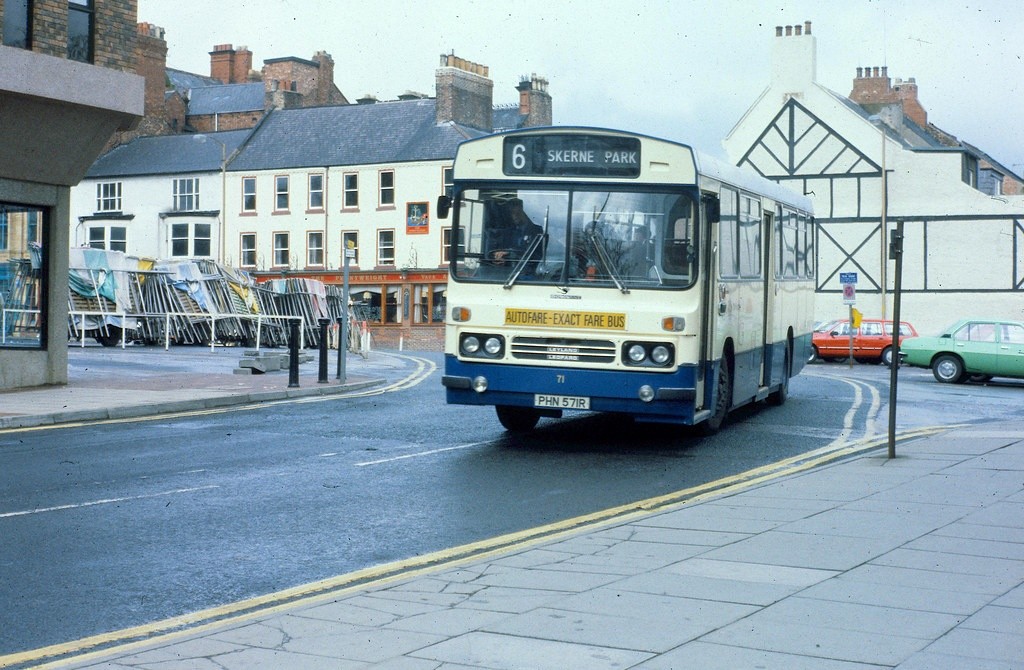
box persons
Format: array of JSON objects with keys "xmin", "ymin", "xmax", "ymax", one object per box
[
  {"xmin": 585, "ymin": 221, "xmax": 656, "ymax": 275},
  {"xmin": 494, "ymin": 199, "xmax": 543, "ymax": 272}
]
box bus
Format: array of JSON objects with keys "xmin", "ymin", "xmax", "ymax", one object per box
[{"xmin": 435, "ymin": 123, "xmax": 817, "ymax": 439}]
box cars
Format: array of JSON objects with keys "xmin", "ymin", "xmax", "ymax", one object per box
[
  {"xmin": 899, "ymin": 319, "xmax": 1024, "ymax": 384},
  {"xmin": 805, "ymin": 317, "xmax": 919, "ymax": 367}
]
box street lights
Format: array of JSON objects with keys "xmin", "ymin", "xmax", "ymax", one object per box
[{"xmin": 868, "ymin": 114, "xmax": 889, "ymax": 318}]
